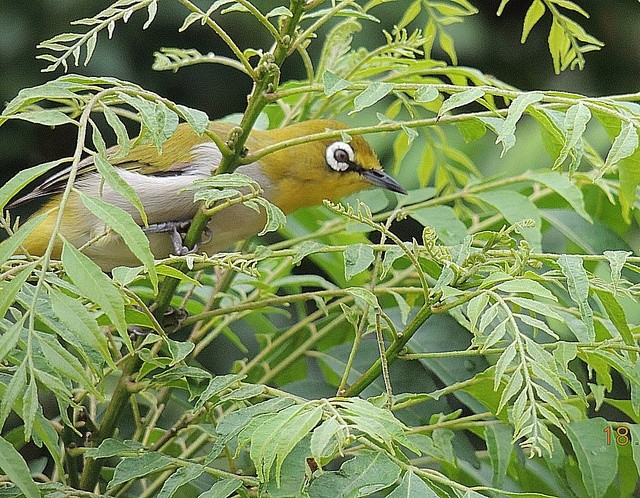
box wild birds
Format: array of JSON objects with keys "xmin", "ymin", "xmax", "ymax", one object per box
[{"xmin": 12, "ymin": 118, "xmax": 413, "ymax": 336}]
